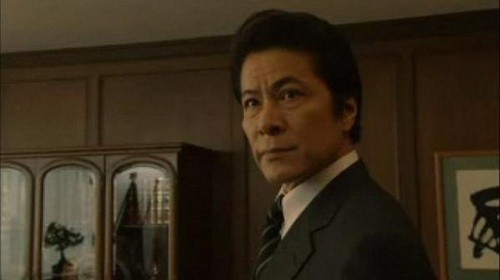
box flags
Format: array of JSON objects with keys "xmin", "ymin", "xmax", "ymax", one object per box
[{"xmin": 114, "ymin": 180, "xmax": 147, "ymax": 280}]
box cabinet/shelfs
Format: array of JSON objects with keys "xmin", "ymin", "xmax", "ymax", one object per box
[{"xmin": 1, "ymin": 142, "xmax": 223, "ymax": 280}]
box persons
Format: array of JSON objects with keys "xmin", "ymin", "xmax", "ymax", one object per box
[{"xmin": 230, "ymin": 9, "xmax": 437, "ymax": 280}]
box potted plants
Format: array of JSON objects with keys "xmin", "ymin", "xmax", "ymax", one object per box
[{"xmin": 44, "ymin": 223, "xmax": 85, "ymax": 280}]
